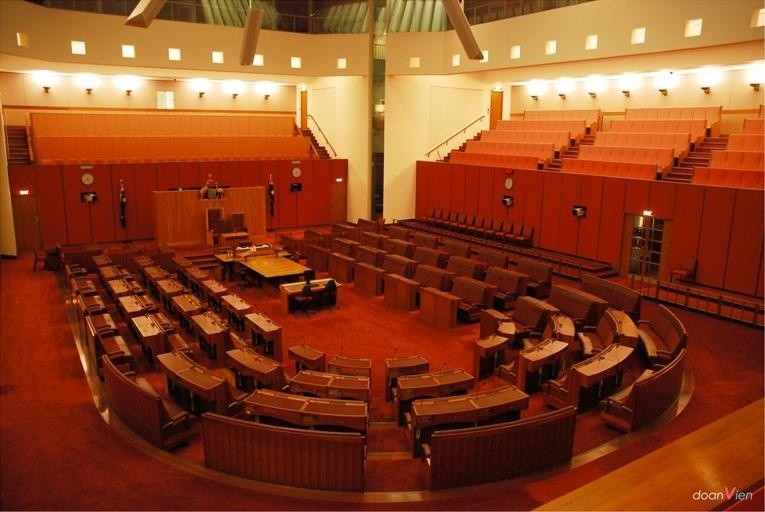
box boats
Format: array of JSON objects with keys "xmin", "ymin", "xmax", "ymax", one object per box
[{"xmin": 290, "ymin": 183, "xmax": 302, "ymax": 192}]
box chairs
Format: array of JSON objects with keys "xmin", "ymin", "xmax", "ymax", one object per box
[{"xmin": 32, "ymin": 242, "xmax": 46, "ymax": 270}]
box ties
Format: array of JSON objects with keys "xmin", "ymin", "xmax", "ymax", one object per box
[{"xmin": 304, "ymin": 269, "xmax": 316, "ymax": 284}]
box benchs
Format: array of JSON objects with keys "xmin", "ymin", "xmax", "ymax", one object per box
[{"xmin": 450, "ymin": 108, "xmax": 765, "ymax": 189}]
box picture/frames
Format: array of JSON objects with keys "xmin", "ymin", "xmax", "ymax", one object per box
[{"xmin": 44, "ymin": 245, "xmax": 58, "ymax": 270}]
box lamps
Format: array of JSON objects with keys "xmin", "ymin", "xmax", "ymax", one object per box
[
  {"xmin": 32, "ymin": 242, "xmax": 46, "ymax": 270},
  {"xmin": 532, "ymin": 82, "xmax": 760, "ymax": 100},
  {"xmin": 43, "ymin": 86, "xmax": 271, "ymax": 100}
]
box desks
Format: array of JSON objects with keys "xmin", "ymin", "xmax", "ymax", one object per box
[
  {"xmin": 183, "ymin": 217, "xmax": 688, "ymax": 331},
  {"xmin": 475, "ymin": 276, "xmax": 688, "ymax": 434},
  {"xmin": 386, "ymin": 356, "xmax": 578, "ymax": 491},
  {"xmin": 44, "ymin": 245, "xmax": 58, "ymax": 270},
  {"xmin": 61, "ymin": 243, "xmax": 283, "ymax": 451},
  {"xmin": 202, "ymin": 342, "xmax": 372, "ymax": 491}
]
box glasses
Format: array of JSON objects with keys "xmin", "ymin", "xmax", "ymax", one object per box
[
  {"xmin": 43, "ymin": 86, "xmax": 271, "ymax": 100},
  {"xmin": 532, "ymin": 82, "xmax": 760, "ymax": 100}
]
